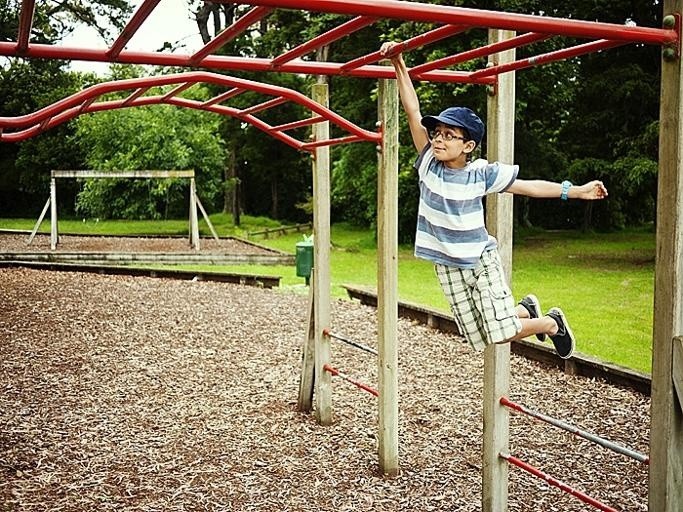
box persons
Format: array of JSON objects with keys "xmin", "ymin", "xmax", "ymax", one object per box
[{"xmin": 379, "ymin": 40, "xmax": 609, "ymax": 360}]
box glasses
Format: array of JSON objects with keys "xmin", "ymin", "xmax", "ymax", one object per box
[{"xmin": 429, "ymin": 130, "xmax": 468, "ymax": 141}]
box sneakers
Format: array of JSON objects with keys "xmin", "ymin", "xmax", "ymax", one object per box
[
  {"xmin": 517, "ymin": 294, "xmax": 548, "ymax": 342},
  {"xmin": 545, "ymin": 307, "xmax": 575, "ymax": 360}
]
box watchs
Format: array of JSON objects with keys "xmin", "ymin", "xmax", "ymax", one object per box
[{"xmin": 560, "ymin": 181, "xmax": 572, "ymax": 200}]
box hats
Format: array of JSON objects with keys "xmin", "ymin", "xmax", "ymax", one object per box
[{"xmin": 421, "ymin": 106, "xmax": 486, "ymax": 146}]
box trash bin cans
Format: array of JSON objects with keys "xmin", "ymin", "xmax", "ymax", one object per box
[{"xmin": 295, "ymin": 242, "xmax": 314, "ymax": 286}]
